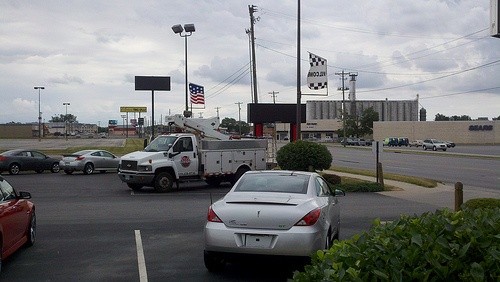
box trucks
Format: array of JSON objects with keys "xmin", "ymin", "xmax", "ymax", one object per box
[{"xmin": 119, "ymin": 134, "xmax": 268, "ymax": 192}]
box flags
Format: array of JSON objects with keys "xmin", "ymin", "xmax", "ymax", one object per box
[
  {"xmin": 307, "ymin": 53, "xmax": 327, "ymax": 90},
  {"xmin": 188, "ymin": 83, "xmax": 205, "ymax": 105}
]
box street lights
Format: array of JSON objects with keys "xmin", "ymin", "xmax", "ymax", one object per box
[
  {"xmin": 172, "ymin": 23, "xmax": 195, "ymax": 110},
  {"xmin": 34, "ymin": 86, "xmax": 46, "ymax": 140},
  {"xmin": 63, "ymin": 103, "xmax": 71, "ymax": 140}
]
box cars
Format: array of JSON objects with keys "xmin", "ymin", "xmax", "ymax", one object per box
[
  {"xmin": 0, "ymin": 148, "xmax": 64, "ymax": 175},
  {"xmin": 340, "ymin": 137, "xmax": 456, "ymax": 151},
  {"xmin": 203, "ymin": 169, "xmax": 345, "ymax": 271},
  {"xmin": 0, "ymin": 176, "xmax": 37, "ymax": 262},
  {"xmin": 59, "ymin": 149, "xmax": 121, "ymax": 175}
]
